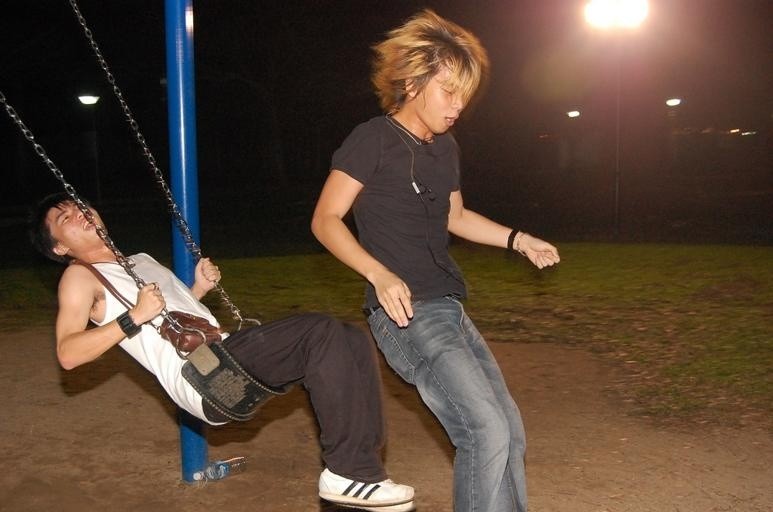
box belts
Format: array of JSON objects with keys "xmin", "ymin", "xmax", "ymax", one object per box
[{"xmin": 361, "ymin": 304, "xmax": 382, "ymax": 320}]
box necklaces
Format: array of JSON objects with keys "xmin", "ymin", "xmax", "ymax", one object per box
[{"xmin": 82, "ymin": 256, "xmax": 135, "ymax": 269}]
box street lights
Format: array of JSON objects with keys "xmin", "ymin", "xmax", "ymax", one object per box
[
  {"xmin": 582, "ymin": 0, "xmax": 647, "ymax": 240},
  {"xmin": 72, "ymin": 77, "xmax": 105, "ymax": 208}
]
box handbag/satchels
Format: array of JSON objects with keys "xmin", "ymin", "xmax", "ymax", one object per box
[{"xmin": 156, "ymin": 309, "xmax": 222, "ymax": 353}]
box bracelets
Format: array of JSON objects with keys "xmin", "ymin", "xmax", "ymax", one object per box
[
  {"xmin": 508, "ymin": 228, "xmax": 518, "ymax": 250},
  {"xmin": 115, "ymin": 309, "xmax": 140, "ymax": 338},
  {"xmin": 517, "ymin": 232, "xmax": 531, "ymax": 256}
]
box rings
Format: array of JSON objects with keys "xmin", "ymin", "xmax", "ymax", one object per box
[{"xmin": 150, "ymin": 282, "xmax": 157, "ymax": 290}]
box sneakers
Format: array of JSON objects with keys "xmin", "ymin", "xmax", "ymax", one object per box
[
  {"xmin": 315, "ymin": 466, "xmax": 416, "ymax": 507},
  {"xmin": 332, "ymin": 500, "xmax": 415, "ymax": 512}
]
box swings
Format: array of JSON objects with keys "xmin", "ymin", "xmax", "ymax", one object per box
[{"xmin": 0, "ymin": 1, "xmax": 293, "ymax": 423}]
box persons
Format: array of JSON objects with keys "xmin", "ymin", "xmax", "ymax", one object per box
[
  {"xmin": 36, "ymin": 195, "xmax": 415, "ymax": 512},
  {"xmin": 311, "ymin": 8, "xmax": 558, "ymax": 512}
]
